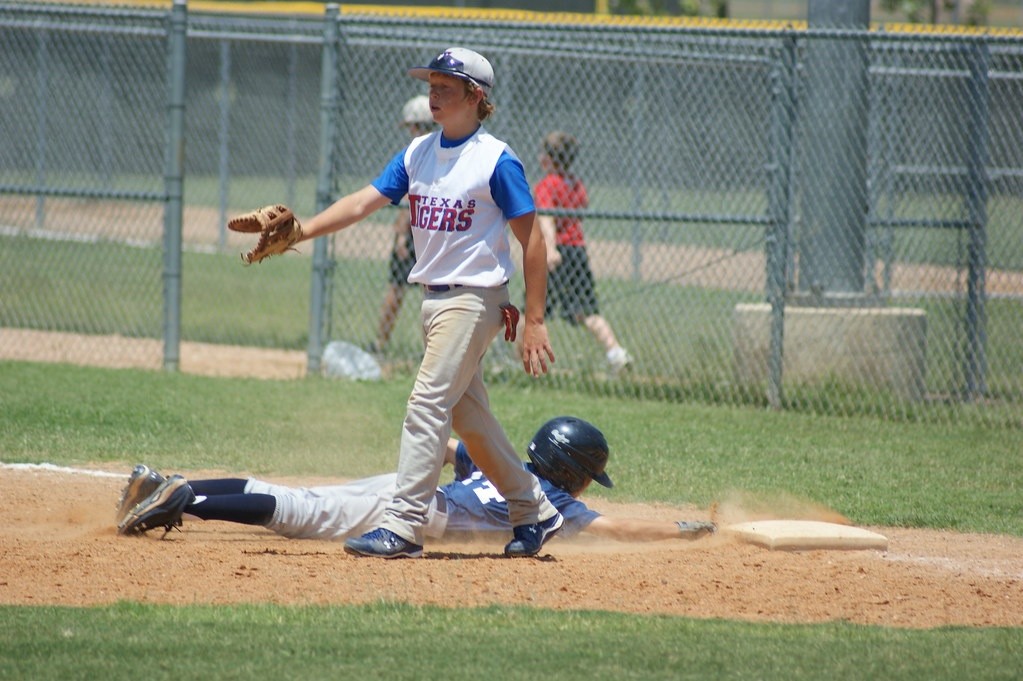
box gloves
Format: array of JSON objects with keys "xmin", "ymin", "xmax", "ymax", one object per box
[{"xmin": 500, "ymin": 304, "xmax": 520, "ymax": 342}]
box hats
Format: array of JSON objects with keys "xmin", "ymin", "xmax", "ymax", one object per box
[
  {"xmin": 396, "ymin": 95, "xmax": 438, "ymax": 130},
  {"xmin": 407, "ymin": 47, "xmax": 494, "ymax": 97}
]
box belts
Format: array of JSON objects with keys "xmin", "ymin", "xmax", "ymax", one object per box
[{"xmin": 423, "ymin": 284, "xmax": 462, "ymax": 292}]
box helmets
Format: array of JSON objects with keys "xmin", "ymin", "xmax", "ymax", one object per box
[{"xmin": 525, "ymin": 416, "xmax": 614, "ymax": 492}]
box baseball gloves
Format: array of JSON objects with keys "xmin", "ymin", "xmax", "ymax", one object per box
[{"xmin": 228, "ymin": 203, "xmax": 303, "ymax": 266}]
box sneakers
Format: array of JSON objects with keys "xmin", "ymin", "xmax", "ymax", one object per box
[
  {"xmin": 343, "ymin": 527, "xmax": 423, "ymax": 559},
  {"xmin": 610, "ymin": 349, "xmax": 634, "ymax": 371},
  {"xmin": 116, "ymin": 474, "xmax": 197, "ymax": 541},
  {"xmin": 369, "ymin": 339, "xmax": 390, "ymax": 353},
  {"xmin": 504, "ymin": 513, "xmax": 566, "ymax": 557},
  {"xmin": 115, "ymin": 464, "xmax": 170, "ymax": 521}
]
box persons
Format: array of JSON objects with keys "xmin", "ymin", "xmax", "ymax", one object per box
[
  {"xmin": 362, "ymin": 95, "xmax": 438, "ymax": 354},
  {"xmin": 115, "ymin": 417, "xmax": 718, "ymax": 539},
  {"xmin": 269, "ymin": 47, "xmax": 563, "ymax": 559},
  {"xmin": 512, "ymin": 132, "xmax": 633, "ymax": 373}
]
